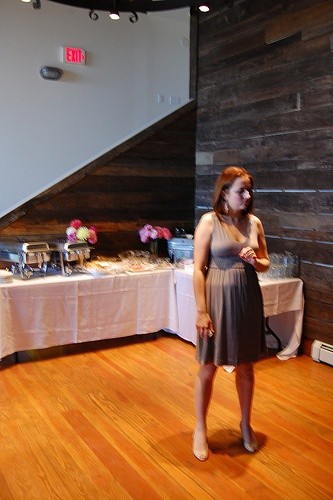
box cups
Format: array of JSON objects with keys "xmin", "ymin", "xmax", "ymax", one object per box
[{"xmin": 262, "ymin": 253, "xmax": 298, "ymax": 280}]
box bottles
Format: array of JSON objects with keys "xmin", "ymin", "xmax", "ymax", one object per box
[{"xmin": 170, "ymin": 250, "xmax": 174, "ymax": 263}]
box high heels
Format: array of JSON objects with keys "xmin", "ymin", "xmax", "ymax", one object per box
[
  {"xmin": 191, "ymin": 430, "xmax": 209, "ymax": 462},
  {"xmin": 240, "ymin": 421, "xmax": 257, "ymax": 454}
]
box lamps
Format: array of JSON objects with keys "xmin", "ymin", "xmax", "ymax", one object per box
[
  {"xmin": 198, "ymin": 0, "xmax": 210, "ymax": 12},
  {"xmin": 108, "ymin": 8, "xmax": 121, "ymax": 20},
  {"xmin": 32, "ymin": 0, "xmax": 41, "ymax": 10},
  {"xmin": 39, "ymin": 65, "xmax": 62, "ymax": 81}
]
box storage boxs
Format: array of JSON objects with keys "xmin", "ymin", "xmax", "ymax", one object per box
[{"xmin": 166, "ymin": 236, "xmax": 193, "ymax": 259}]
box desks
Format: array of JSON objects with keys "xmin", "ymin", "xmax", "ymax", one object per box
[{"xmin": 0, "ymin": 266, "xmax": 305, "ymax": 373}]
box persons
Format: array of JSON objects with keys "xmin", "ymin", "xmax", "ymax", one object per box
[{"xmin": 192, "ymin": 167, "xmax": 271, "ymax": 462}]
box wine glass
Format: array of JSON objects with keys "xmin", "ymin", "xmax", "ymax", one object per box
[{"xmin": 117, "ymin": 250, "xmax": 151, "ymax": 272}]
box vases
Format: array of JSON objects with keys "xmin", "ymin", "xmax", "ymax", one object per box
[{"xmin": 147, "ymin": 238, "xmax": 158, "ymax": 256}]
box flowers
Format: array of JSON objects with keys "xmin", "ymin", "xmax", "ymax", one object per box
[
  {"xmin": 139, "ymin": 223, "xmax": 174, "ymax": 242},
  {"xmin": 65, "ymin": 219, "xmax": 98, "ymax": 245}
]
